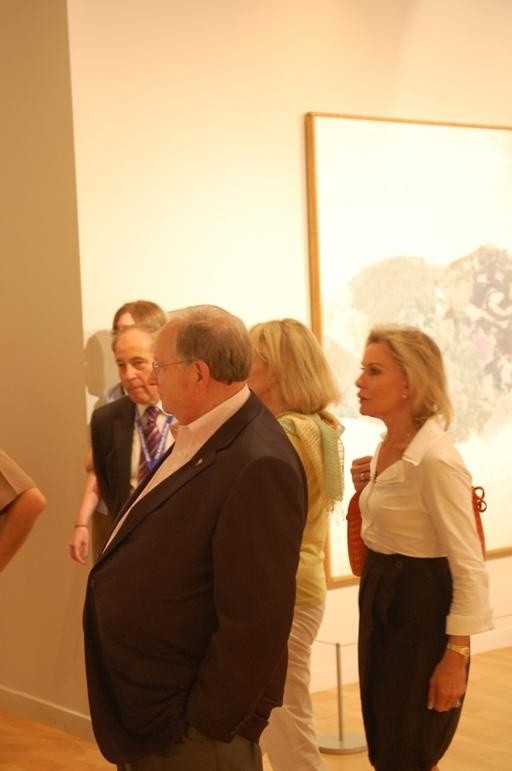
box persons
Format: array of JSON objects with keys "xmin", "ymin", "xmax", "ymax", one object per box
[
  {"xmin": 67, "ymin": 298, "xmax": 167, "ymax": 566},
  {"xmin": 244, "ymin": 316, "xmax": 348, "ymax": 770},
  {"xmin": 349, "ymin": 320, "xmax": 498, "ymax": 771},
  {"xmin": 80, "ymin": 304, "xmax": 313, "ymax": 771},
  {"xmin": 90, "ymin": 321, "xmax": 181, "ymax": 521},
  {"xmin": 0, "ymin": 445, "xmax": 47, "ymax": 572}
]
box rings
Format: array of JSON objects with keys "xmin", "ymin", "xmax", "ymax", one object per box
[
  {"xmin": 454, "ymin": 700, "xmax": 461, "ymax": 708},
  {"xmin": 360, "ymin": 472, "xmax": 365, "ymax": 480}
]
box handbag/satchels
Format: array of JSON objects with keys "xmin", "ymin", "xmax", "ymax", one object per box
[{"xmin": 344, "ymin": 476, "xmax": 488, "ymax": 578}]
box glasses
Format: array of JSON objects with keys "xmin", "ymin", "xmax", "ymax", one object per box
[{"xmin": 146, "ymin": 356, "xmax": 200, "ymax": 378}]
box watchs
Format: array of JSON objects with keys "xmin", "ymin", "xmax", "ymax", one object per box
[{"xmin": 446, "ymin": 641, "xmax": 471, "ymax": 659}]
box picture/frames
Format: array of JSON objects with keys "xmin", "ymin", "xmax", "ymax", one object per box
[{"xmin": 304, "ymin": 109, "xmax": 512, "ymax": 589}]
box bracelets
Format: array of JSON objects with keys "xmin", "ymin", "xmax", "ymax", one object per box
[{"xmin": 74, "ymin": 524, "xmax": 89, "ymax": 529}]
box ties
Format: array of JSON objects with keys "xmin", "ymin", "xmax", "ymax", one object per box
[{"xmin": 138, "ymin": 405, "xmax": 168, "ymax": 491}]
